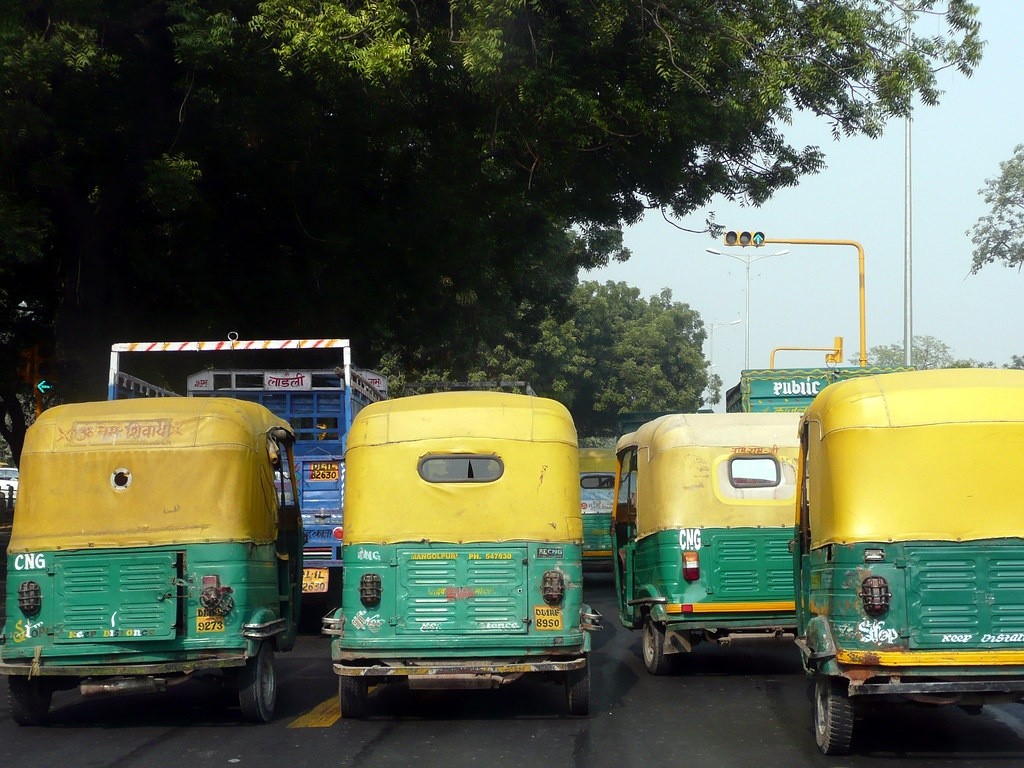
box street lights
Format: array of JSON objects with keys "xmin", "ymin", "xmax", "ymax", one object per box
[{"xmin": 706, "ymin": 247, "xmax": 793, "ymax": 371}]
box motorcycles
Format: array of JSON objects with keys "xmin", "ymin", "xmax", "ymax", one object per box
[
  {"xmin": 616, "ymin": 411, "xmax": 807, "ymax": 676},
  {"xmin": 797, "ymin": 366, "xmax": 1024, "ymax": 755},
  {"xmin": 344, "ymin": 391, "xmax": 602, "ymax": 720},
  {"xmin": 578, "ymin": 445, "xmax": 628, "ymax": 571},
  {"xmin": 3, "ymin": 396, "xmax": 311, "ymax": 724}
]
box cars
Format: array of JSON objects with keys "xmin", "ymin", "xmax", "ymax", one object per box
[{"xmin": 0, "ymin": 468, "xmax": 19, "ymax": 510}]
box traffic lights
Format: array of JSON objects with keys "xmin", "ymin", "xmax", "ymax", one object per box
[{"xmin": 723, "ymin": 230, "xmax": 765, "ymax": 246}]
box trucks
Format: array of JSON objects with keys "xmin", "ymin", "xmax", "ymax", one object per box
[
  {"xmin": 102, "ymin": 332, "xmax": 393, "ymax": 601},
  {"xmin": 724, "ymin": 357, "xmax": 935, "ymax": 416}
]
